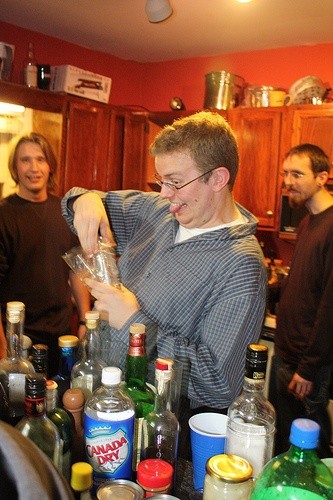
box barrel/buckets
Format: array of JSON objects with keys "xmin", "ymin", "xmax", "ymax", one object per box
[{"xmin": 204, "ymin": 72, "xmax": 244, "ymax": 111}]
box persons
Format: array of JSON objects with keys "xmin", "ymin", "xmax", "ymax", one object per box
[
  {"xmin": 0, "ymin": 131, "xmax": 91, "ymax": 395},
  {"xmin": 268, "ymin": 142, "xmax": 333, "ymax": 459},
  {"xmin": 61, "ymin": 110, "xmax": 268, "ymax": 464}
]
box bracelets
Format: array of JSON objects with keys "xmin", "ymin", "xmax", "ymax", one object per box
[{"xmin": 78, "ymin": 320, "xmax": 85, "ymax": 325}]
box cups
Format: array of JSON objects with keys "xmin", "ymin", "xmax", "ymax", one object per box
[
  {"xmin": 0, "ymin": 41, "xmax": 15, "ymax": 81},
  {"xmin": 268, "ymin": 90, "xmax": 292, "ymax": 107},
  {"xmin": 36, "ymin": 64, "xmax": 51, "ymax": 90},
  {"xmin": 188, "ymin": 413, "xmax": 229, "ymax": 495}
]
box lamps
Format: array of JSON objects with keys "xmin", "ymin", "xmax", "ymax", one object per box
[{"xmin": 146, "ymin": 0, "xmax": 172, "ymax": 23}]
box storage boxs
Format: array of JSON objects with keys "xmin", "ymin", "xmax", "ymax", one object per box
[{"xmin": 49, "ymin": 65, "xmax": 112, "ymax": 104}]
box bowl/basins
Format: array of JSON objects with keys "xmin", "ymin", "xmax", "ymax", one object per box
[{"xmin": 244, "ymin": 85, "xmax": 272, "ymax": 107}]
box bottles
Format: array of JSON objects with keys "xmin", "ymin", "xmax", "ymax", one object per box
[
  {"xmin": 81, "ymin": 366, "xmax": 136, "ymax": 491},
  {"xmin": 122, "ymin": 324, "xmax": 158, "ymax": 484},
  {"xmin": 0, "ymin": 300, "xmax": 105, "ymax": 479},
  {"xmin": 68, "ymin": 462, "xmax": 98, "ymax": 500},
  {"xmin": 22, "ymin": 41, "xmax": 38, "ymax": 89},
  {"xmin": 247, "ymin": 418, "xmax": 333, "ymax": 500},
  {"xmin": 139, "ymin": 358, "xmax": 180, "ymax": 493},
  {"xmin": 223, "ymin": 343, "xmax": 277, "ymax": 483},
  {"xmin": 135, "ymin": 458, "xmax": 174, "ymax": 499}
]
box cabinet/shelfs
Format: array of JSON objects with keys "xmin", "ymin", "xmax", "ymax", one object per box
[{"xmin": 0, "ymin": 80, "xmax": 333, "ymax": 239}]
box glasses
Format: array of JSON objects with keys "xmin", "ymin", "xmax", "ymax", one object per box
[
  {"xmin": 279, "ymin": 171, "xmax": 320, "ymax": 181},
  {"xmin": 153, "ymin": 167, "xmax": 220, "ymax": 192}
]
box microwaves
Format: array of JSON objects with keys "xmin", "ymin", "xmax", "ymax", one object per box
[{"xmin": 278, "ymin": 178, "xmax": 333, "ymax": 240}]
